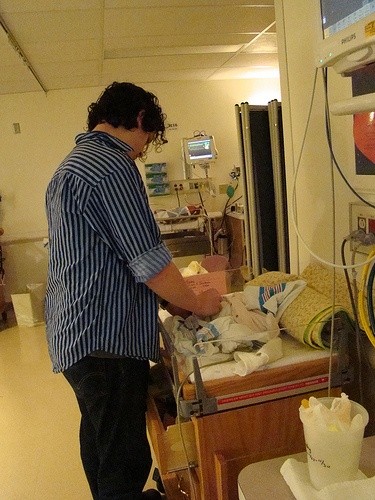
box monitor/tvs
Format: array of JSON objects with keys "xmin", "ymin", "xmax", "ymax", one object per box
[
  {"xmin": 314, "ymin": 0, "xmax": 375, "ymax": 75},
  {"xmin": 183, "ymin": 136, "xmax": 217, "ymax": 164}
]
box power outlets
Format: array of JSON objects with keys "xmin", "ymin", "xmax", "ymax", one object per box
[
  {"xmin": 171, "ymin": 180, "xmax": 213, "ymax": 195},
  {"xmin": 348, "ymin": 202, "xmax": 375, "ymax": 255}
]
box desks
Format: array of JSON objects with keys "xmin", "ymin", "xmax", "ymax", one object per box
[{"xmin": 238, "ymin": 435, "xmax": 375, "ymax": 500}]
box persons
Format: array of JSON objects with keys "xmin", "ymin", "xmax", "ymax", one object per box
[{"xmin": 46, "ymin": 82, "xmax": 222, "ymax": 500}]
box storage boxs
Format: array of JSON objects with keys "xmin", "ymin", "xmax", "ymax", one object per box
[{"xmin": 183, "ymin": 269, "xmax": 244, "ymax": 301}]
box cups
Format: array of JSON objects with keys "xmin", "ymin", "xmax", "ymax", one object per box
[{"xmin": 298, "ymin": 396, "xmax": 369, "ymax": 490}]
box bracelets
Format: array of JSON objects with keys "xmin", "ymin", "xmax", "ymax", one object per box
[{"xmin": 160, "ymin": 300, "xmax": 170, "ymax": 310}]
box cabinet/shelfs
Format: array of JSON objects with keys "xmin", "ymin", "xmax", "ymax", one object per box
[{"xmin": 139, "ymin": 287, "xmax": 355, "ymax": 495}]
